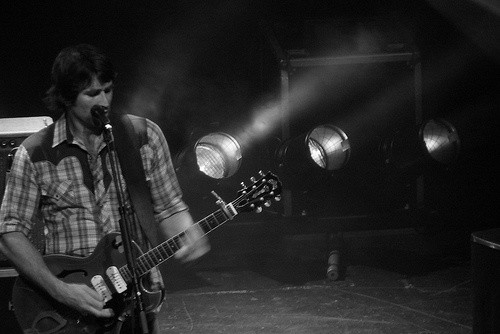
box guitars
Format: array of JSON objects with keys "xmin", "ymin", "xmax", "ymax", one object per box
[{"xmin": 1, "ymin": 168, "xmax": 286, "ymax": 334}]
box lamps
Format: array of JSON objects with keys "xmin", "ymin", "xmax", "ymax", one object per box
[
  {"xmin": 379, "ymin": 117, "xmax": 461, "ymax": 179},
  {"xmin": 172, "ymin": 131, "xmax": 242, "ymax": 208},
  {"xmin": 273, "ymin": 124, "xmax": 350, "ymax": 193}
]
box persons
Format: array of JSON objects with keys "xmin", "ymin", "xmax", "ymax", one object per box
[{"xmin": 0, "ymin": 42, "xmax": 210, "ymax": 334}]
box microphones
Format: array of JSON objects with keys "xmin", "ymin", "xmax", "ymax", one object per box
[{"xmin": 90, "ymin": 105, "xmax": 108, "ymax": 119}]
